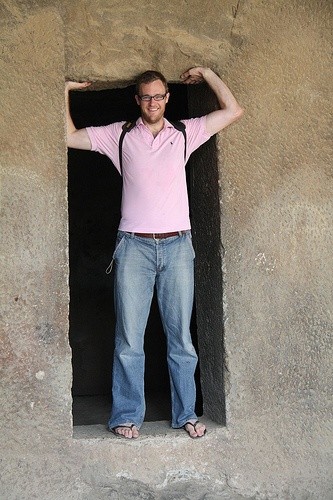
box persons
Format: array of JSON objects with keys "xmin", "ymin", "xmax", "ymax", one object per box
[{"xmin": 58, "ymin": 65, "xmax": 246, "ymax": 440}]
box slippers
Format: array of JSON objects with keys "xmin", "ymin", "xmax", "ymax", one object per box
[
  {"xmin": 112, "ymin": 423, "xmax": 137, "ymax": 439},
  {"xmin": 183, "ymin": 419, "xmax": 207, "ymax": 438}
]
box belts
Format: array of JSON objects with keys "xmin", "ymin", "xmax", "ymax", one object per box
[{"xmin": 127, "ymin": 232, "xmax": 185, "ymax": 239}]
